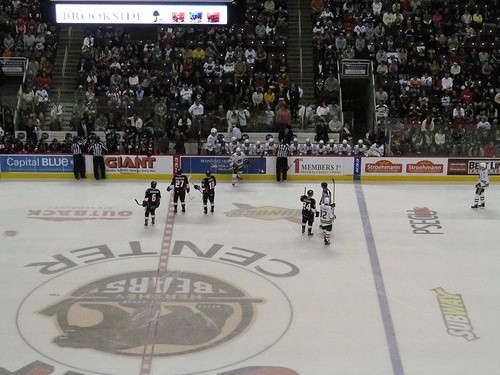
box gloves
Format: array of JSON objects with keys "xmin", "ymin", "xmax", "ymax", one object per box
[
  {"xmin": 143, "ymin": 201, "xmax": 147, "ymax": 207},
  {"xmin": 194, "ymin": 185, "xmax": 200, "ymax": 189},
  {"xmin": 331, "ymin": 203, "xmax": 335, "ymax": 208},
  {"xmin": 315, "ymin": 211, "xmax": 319, "ymax": 217},
  {"xmin": 167, "ymin": 186, "xmax": 171, "ymax": 192}
]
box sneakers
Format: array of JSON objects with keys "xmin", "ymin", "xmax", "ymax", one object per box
[
  {"xmin": 204, "ymin": 209, "xmax": 215, "ymax": 214},
  {"xmin": 144, "ymin": 222, "xmax": 155, "ymax": 226},
  {"xmin": 324, "ymin": 238, "xmax": 331, "ymax": 246},
  {"xmin": 479, "ymin": 202, "xmax": 485, "ymax": 208},
  {"xmin": 302, "ymin": 225, "xmax": 314, "ymax": 237},
  {"xmin": 471, "ymin": 205, "xmax": 478, "ymax": 209}
]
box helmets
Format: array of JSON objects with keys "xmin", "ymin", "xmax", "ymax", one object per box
[
  {"xmin": 479, "ymin": 163, "xmax": 487, "ymax": 169},
  {"xmin": 206, "ymin": 170, "xmax": 211, "ymax": 174},
  {"xmin": 210, "ymin": 127, "xmax": 274, "ymax": 146},
  {"xmin": 151, "ymin": 181, "xmax": 157, "ymax": 188},
  {"xmin": 324, "ymin": 197, "xmax": 330, "ymax": 204},
  {"xmin": 292, "ymin": 137, "xmax": 364, "ymax": 143},
  {"xmin": 307, "ymin": 190, "xmax": 313, "ymax": 196},
  {"xmin": 177, "ymin": 168, "xmax": 182, "ymax": 175},
  {"xmin": 235, "ymin": 147, "xmax": 242, "ymax": 152}
]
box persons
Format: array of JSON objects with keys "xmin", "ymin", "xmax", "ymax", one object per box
[
  {"xmin": 320, "ymin": 197, "xmax": 337, "ymax": 245},
  {"xmin": 143, "ymin": 181, "xmax": 161, "ymax": 225},
  {"xmin": 230, "ymin": 147, "xmax": 249, "ymax": 185},
  {"xmin": 471, "ymin": 163, "xmax": 489, "ymax": 208},
  {"xmin": 300, "ymin": 190, "xmax": 319, "ymax": 236},
  {"xmin": 71, "ymin": 136, "xmax": 86, "ymax": 179},
  {"xmin": 0, "ymin": 0, "xmax": 500, "ymax": 156},
  {"xmin": 195, "ymin": 170, "xmax": 216, "ymax": 214},
  {"xmin": 276, "ymin": 138, "xmax": 291, "ymax": 182},
  {"xmin": 167, "ymin": 168, "xmax": 190, "ymax": 213},
  {"xmin": 319, "ymin": 182, "xmax": 331, "ymax": 205},
  {"xmin": 89, "ymin": 136, "xmax": 108, "ymax": 180}
]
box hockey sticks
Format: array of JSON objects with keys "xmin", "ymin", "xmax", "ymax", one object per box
[
  {"xmin": 190, "ymin": 180, "xmax": 207, "ymax": 195},
  {"xmin": 304, "ymin": 187, "xmax": 306, "ymax": 195},
  {"xmin": 332, "ymin": 140, "xmax": 337, "ymax": 154},
  {"xmin": 135, "ymin": 198, "xmax": 148, "ymax": 206},
  {"xmin": 332, "ymin": 178, "xmax": 335, "ymax": 223}
]
